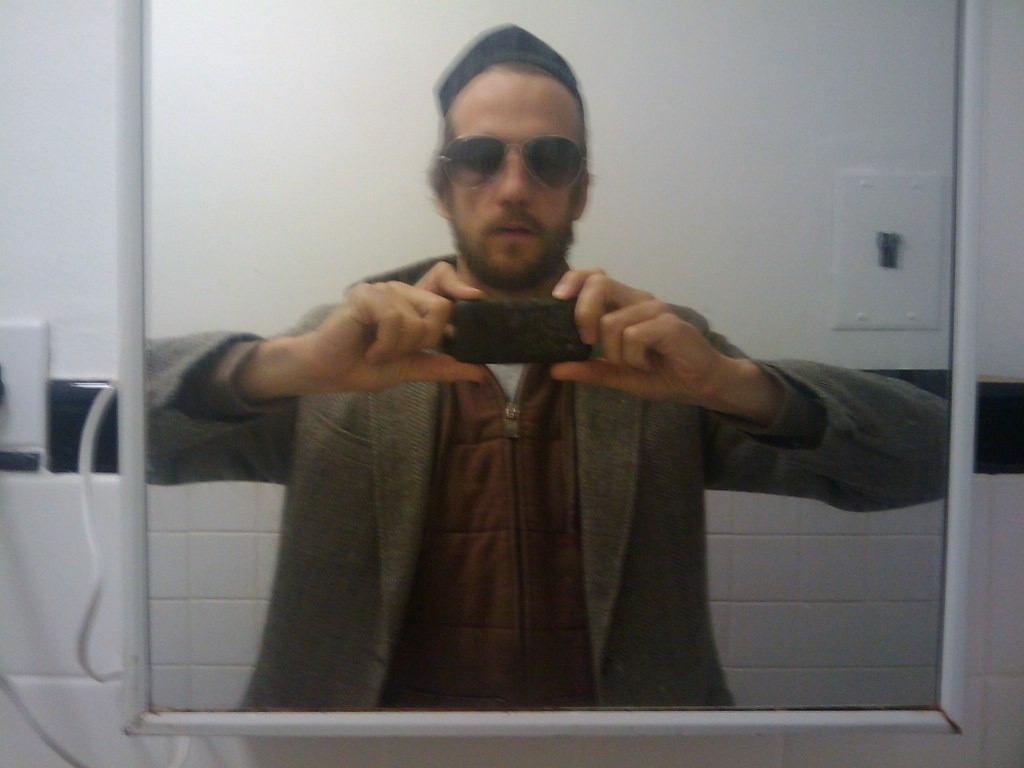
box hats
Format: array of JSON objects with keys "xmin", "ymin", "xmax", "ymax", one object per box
[{"xmin": 436, "ymin": 23, "xmax": 578, "ymax": 117}]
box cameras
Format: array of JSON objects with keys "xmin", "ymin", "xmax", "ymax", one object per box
[{"xmin": 441, "ymin": 293, "xmax": 596, "ymax": 365}]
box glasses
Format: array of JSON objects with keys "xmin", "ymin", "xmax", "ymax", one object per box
[{"xmin": 438, "ymin": 133, "xmax": 586, "ymax": 191}]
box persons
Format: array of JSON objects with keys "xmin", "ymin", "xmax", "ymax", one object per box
[{"xmin": 142, "ymin": 26, "xmax": 951, "ymax": 713}]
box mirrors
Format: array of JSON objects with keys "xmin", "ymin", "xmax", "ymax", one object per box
[{"xmin": 111, "ymin": 0, "xmax": 983, "ymax": 740}]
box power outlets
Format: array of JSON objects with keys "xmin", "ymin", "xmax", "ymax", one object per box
[{"xmin": 0, "ymin": 325, "xmax": 56, "ymax": 451}]
